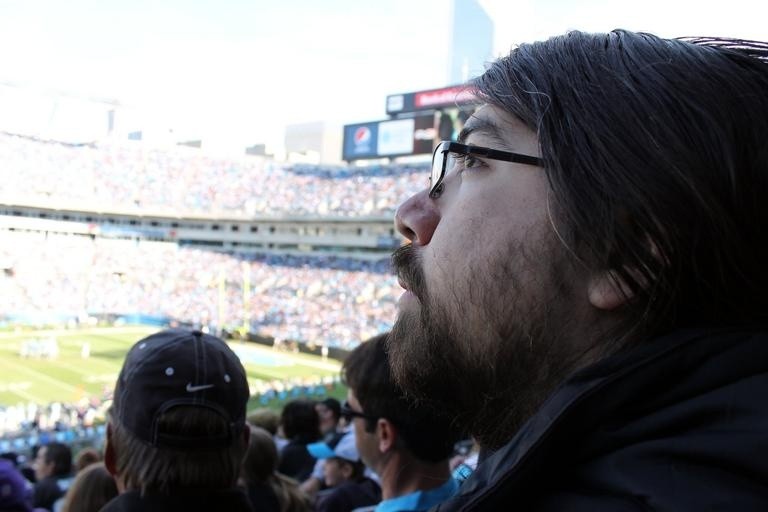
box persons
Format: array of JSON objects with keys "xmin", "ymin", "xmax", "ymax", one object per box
[
  {"xmin": 2, "ymin": 110, "xmax": 487, "ymax": 512},
  {"xmin": 394, "ymin": 28, "xmax": 768, "ymax": 512}
]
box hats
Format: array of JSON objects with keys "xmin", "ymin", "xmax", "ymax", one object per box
[
  {"xmin": 306, "ymin": 429, "xmax": 360, "ymax": 462},
  {"xmin": 113, "ymin": 329, "xmax": 249, "ymax": 444}
]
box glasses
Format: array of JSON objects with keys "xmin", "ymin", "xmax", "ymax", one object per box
[
  {"xmin": 342, "ymin": 400, "xmax": 377, "ymax": 424},
  {"xmin": 429, "ymin": 140, "xmax": 547, "ymax": 197}
]
